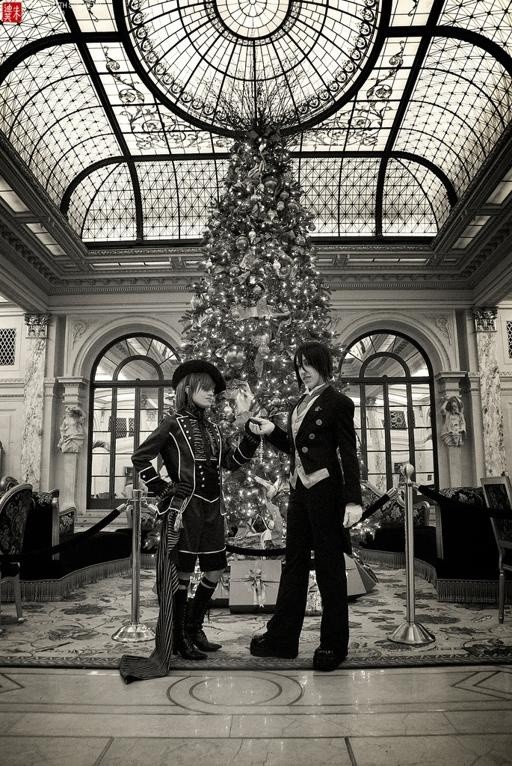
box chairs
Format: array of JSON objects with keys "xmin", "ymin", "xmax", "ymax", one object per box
[
  {"xmin": 0, "ymin": 483, "xmax": 33, "ymax": 624},
  {"xmin": 479, "ymin": 476, "xmax": 512, "ymax": 626}
]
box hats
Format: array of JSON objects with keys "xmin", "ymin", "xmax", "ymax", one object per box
[{"xmin": 171, "ymin": 360, "xmax": 226, "ymax": 395}]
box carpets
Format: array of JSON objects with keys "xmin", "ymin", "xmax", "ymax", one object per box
[{"xmin": 0, "ymin": 568, "xmax": 512, "ymax": 670}]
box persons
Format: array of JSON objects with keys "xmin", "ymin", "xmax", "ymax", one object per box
[
  {"xmin": 117, "ymin": 358, "xmax": 262, "ymax": 686},
  {"xmin": 248, "ymin": 342, "xmax": 364, "ymax": 670}
]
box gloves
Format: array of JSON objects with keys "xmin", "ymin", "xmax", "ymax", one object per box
[
  {"xmin": 245, "ymin": 418, "xmax": 261, "ymax": 442},
  {"xmin": 341, "ymin": 504, "xmax": 363, "ymax": 528},
  {"xmin": 249, "ymin": 416, "xmax": 274, "ymax": 436},
  {"xmin": 167, "ymin": 482, "xmax": 192, "ymax": 497}
]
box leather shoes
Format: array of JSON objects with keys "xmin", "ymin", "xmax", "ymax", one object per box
[
  {"xmin": 311, "ymin": 643, "xmax": 348, "ymax": 671},
  {"xmin": 249, "ymin": 634, "xmax": 299, "ymax": 659}
]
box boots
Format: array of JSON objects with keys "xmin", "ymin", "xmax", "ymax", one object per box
[
  {"xmin": 172, "ymin": 602, "xmax": 208, "ymax": 659},
  {"xmin": 185, "ymin": 598, "xmax": 222, "ymax": 650}
]
